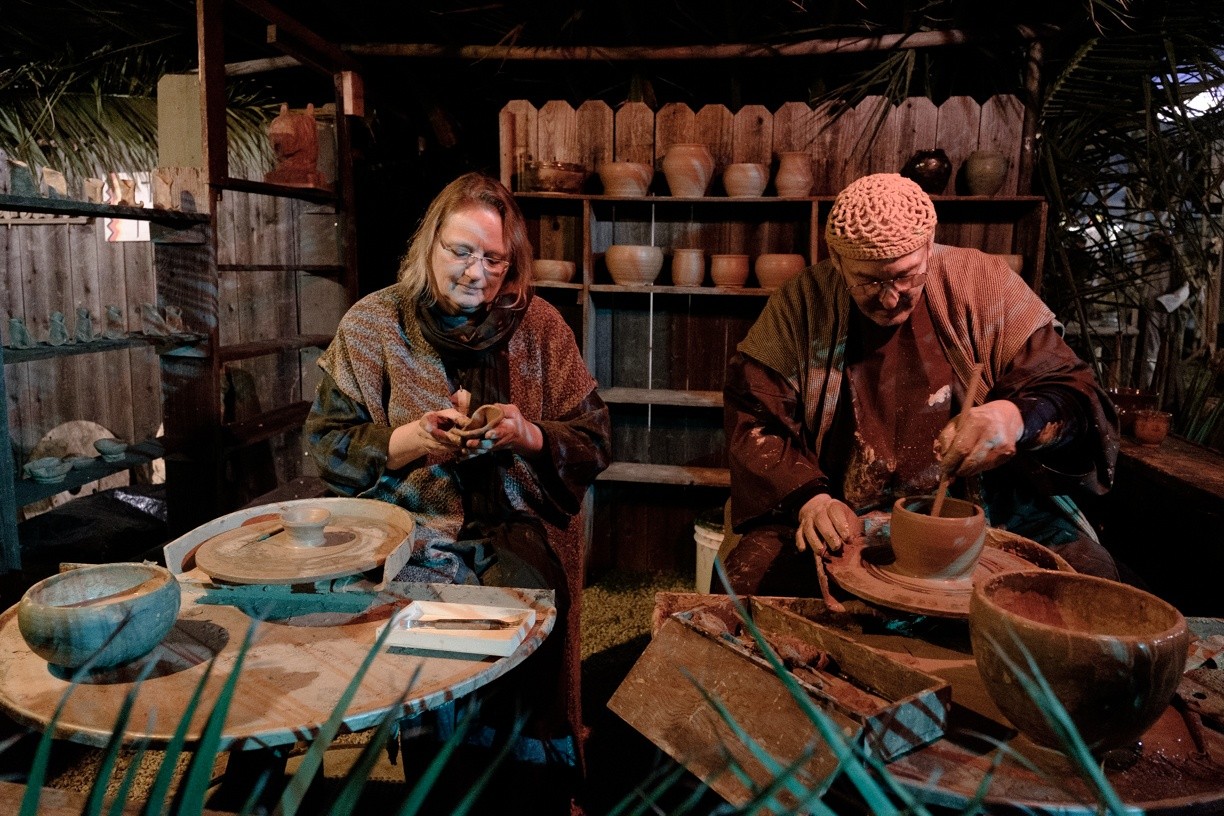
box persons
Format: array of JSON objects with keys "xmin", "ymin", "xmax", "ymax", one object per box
[
  {"xmin": 304, "ymin": 173, "xmax": 612, "ymax": 816},
  {"xmin": 710, "ymin": 173, "xmax": 1121, "ymax": 596},
  {"xmin": 1123, "ymin": 233, "xmax": 1199, "ymax": 436}
]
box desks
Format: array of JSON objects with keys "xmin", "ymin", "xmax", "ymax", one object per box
[
  {"xmin": 1118, "ymin": 426, "xmax": 1224, "ymax": 498},
  {"xmin": 0, "ymin": 568, "xmax": 555, "ymax": 816}
]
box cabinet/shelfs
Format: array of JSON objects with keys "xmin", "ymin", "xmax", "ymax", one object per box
[
  {"xmin": 0, "ymin": 195, "xmax": 213, "ymax": 578},
  {"xmin": 500, "ymin": 96, "xmax": 1050, "ymax": 558},
  {"xmin": 151, "ymin": 167, "xmax": 360, "ymax": 535}
]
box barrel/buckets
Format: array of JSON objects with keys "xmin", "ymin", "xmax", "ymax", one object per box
[{"xmin": 693, "ymin": 523, "xmax": 725, "ymax": 595}]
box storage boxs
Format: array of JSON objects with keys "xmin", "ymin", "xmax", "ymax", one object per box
[{"xmin": 608, "ymin": 596, "xmax": 952, "ymax": 815}]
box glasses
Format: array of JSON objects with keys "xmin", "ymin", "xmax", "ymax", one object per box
[
  {"xmin": 438, "ymin": 234, "xmax": 512, "ymax": 277},
  {"xmin": 839, "ymin": 264, "xmax": 929, "ymax": 297}
]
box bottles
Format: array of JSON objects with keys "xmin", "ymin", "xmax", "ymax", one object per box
[
  {"xmin": 909, "ymin": 148, "xmax": 952, "ymax": 197},
  {"xmin": 671, "ymin": 248, "xmax": 705, "ymax": 287},
  {"xmin": 724, "ymin": 163, "xmax": 770, "ymax": 198},
  {"xmin": 662, "ymin": 143, "xmax": 714, "ymax": 198},
  {"xmin": 966, "ymin": 149, "xmax": 1008, "ymax": 195},
  {"xmin": 775, "ymin": 151, "xmax": 815, "ymax": 198}
]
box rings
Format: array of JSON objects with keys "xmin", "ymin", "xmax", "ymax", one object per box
[{"xmin": 989, "ymin": 440, "xmax": 995, "ymax": 450}]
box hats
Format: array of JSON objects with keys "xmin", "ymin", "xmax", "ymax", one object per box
[{"xmin": 826, "ymin": 173, "xmax": 937, "ymax": 260}]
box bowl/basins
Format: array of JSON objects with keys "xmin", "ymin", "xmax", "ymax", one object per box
[
  {"xmin": 64, "ymin": 457, "xmax": 94, "ymax": 470},
  {"xmin": 532, "ymin": 259, "xmax": 575, "ymax": 283},
  {"xmin": 755, "ymin": 253, "xmax": 806, "ymax": 290},
  {"xmin": 599, "ymin": 162, "xmax": 653, "ymax": 197},
  {"xmin": 889, "ymin": 494, "xmax": 986, "ymax": 581},
  {"xmin": 969, "ymin": 568, "xmax": 1189, "ymax": 752},
  {"xmin": 1135, "ymin": 409, "xmax": 1172, "ymax": 447},
  {"xmin": 29, "ymin": 463, "xmax": 72, "ymax": 482},
  {"xmin": 17, "ymin": 562, "xmax": 181, "ymax": 687},
  {"xmin": 711, "ymin": 255, "xmax": 750, "ymax": 289},
  {"xmin": 536, "ymin": 161, "xmax": 586, "ymax": 193},
  {"xmin": 95, "ymin": 438, "xmax": 128, "ymax": 462},
  {"xmin": 605, "ymin": 245, "xmax": 663, "ymax": 285}
]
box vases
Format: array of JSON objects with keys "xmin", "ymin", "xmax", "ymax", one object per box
[
  {"xmin": 970, "ymin": 569, "xmax": 1189, "ymax": 751},
  {"xmin": 523, "ymin": 161, "xmax": 588, "ymax": 192},
  {"xmin": 710, "ymin": 254, "xmax": 750, "ymax": 288},
  {"xmin": 18, "ymin": 562, "xmax": 181, "ymax": 669},
  {"xmin": 663, "ymin": 143, "xmax": 715, "ymax": 197},
  {"xmin": 595, "ymin": 161, "xmax": 656, "ymax": 197},
  {"xmin": 776, "ymin": 151, "xmax": 814, "ymax": 198},
  {"xmin": 673, "ymin": 249, "xmax": 705, "ymax": 285},
  {"xmin": 722, "ymin": 162, "xmax": 769, "ymax": 197},
  {"xmin": 755, "ymin": 254, "xmax": 806, "ymax": 290},
  {"xmin": 890, "ymin": 496, "xmax": 986, "ymax": 579},
  {"xmin": 606, "ymin": 245, "xmax": 664, "ymax": 286},
  {"xmin": 1134, "ymin": 411, "xmax": 1173, "ymax": 447},
  {"xmin": 1106, "ymin": 387, "xmax": 1160, "ymax": 432},
  {"xmin": 533, "ymin": 259, "xmax": 576, "ymax": 282}
]
